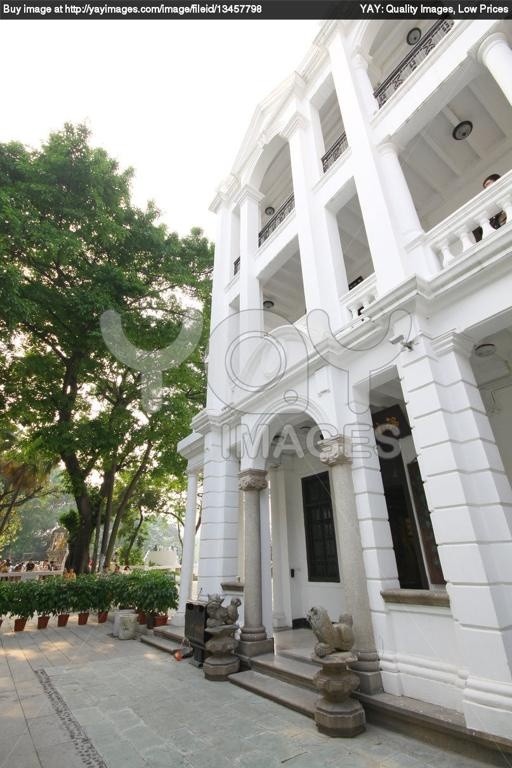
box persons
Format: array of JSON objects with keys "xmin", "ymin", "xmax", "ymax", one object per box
[
  {"xmin": 68, "ymin": 568, "xmax": 76, "ymax": 580},
  {"xmin": 0, "ymin": 559, "xmax": 58, "ymax": 582},
  {"xmin": 63, "ymin": 568, "xmax": 69, "ymax": 578},
  {"xmin": 101, "ymin": 566, "xmax": 130, "ymax": 573}
]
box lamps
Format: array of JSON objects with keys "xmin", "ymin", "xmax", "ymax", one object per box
[
  {"xmin": 475, "ymin": 344, "xmax": 497, "ymax": 358},
  {"xmin": 265, "ymin": 207, "xmax": 275, "ymax": 216},
  {"xmin": 452, "ymin": 120, "xmax": 473, "ymax": 140},
  {"xmin": 273, "ymin": 435, "xmax": 285, "ymax": 443},
  {"xmin": 263, "ymin": 300, "xmax": 274, "ymax": 308},
  {"xmin": 406, "ymin": 28, "xmax": 422, "ymax": 45}
]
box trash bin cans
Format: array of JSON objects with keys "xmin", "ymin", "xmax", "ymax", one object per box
[{"xmin": 184, "ymin": 601, "xmax": 212, "ymax": 668}]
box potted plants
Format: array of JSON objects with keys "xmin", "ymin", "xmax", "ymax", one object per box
[{"xmin": 0, "ymin": 570, "xmax": 180, "ymax": 634}]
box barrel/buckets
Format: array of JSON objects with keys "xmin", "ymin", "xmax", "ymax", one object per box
[
  {"xmin": 112, "ymin": 609, "xmax": 135, "ymax": 636},
  {"xmin": 118, "ymin": 614, "xmax": 139, "ymax": 640}
]
